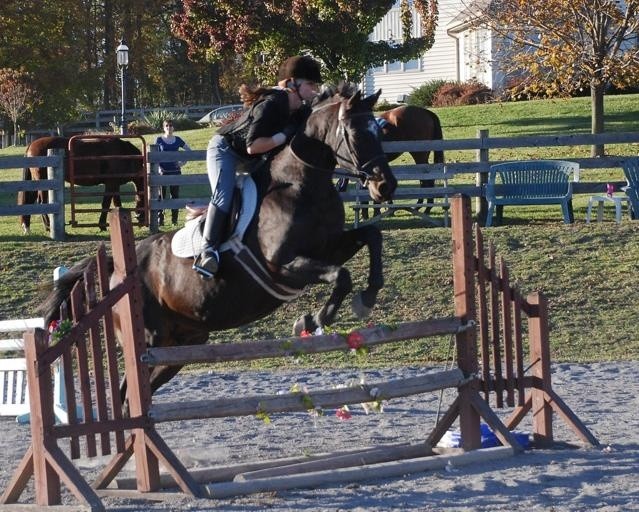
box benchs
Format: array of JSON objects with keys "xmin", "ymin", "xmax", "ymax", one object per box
[
  {"xmin": 620, "ymin": 156, "xmax": 639, "ymax": 219},
  {"xmin": 485, "ymin": 159, "xmax": 580, "ymax": 226}
]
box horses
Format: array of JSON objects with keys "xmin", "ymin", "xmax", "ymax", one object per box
[
  {"xmin": 18, "ymin": 135, "xmax": 145, "ymax": 235},
  {"xmin": 24, "ymin": 89, "xmax": 398, "ymax": 421},
  {"xmin": 359, "ymin": 104, "xmax": 445, "ymax": 223}
]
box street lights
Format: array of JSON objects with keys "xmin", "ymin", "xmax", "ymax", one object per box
[{"xmin": 116, "ymin": 36, "xmax": 130, "ymax": 137}]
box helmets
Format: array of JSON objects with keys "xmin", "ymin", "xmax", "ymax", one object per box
[{"xmin": 277, "ymin": 55, "xmax": 325, "ymax": 83}]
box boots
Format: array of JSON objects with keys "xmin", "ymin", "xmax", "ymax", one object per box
[{"xmin": 200, "ymin": 202, "xmax": 230, "ymax": 281}]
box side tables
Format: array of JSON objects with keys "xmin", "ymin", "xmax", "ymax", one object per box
[{"xmin": 586, "ymin": 196, "xmax": 632, "ymax": 223}]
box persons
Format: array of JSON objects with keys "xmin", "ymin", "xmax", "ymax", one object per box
[
  {"xmin": 154, "ymin": 119, "xmax": 192, "ymax": 227},
  {"xmin": 194, "ymin": 55, "xmax": 325, "ymax": 282}
]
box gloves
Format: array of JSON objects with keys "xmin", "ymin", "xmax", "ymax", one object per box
[{"xmin": 289, "ymin": 105, "xmax": 312, "ymax": 129}]
